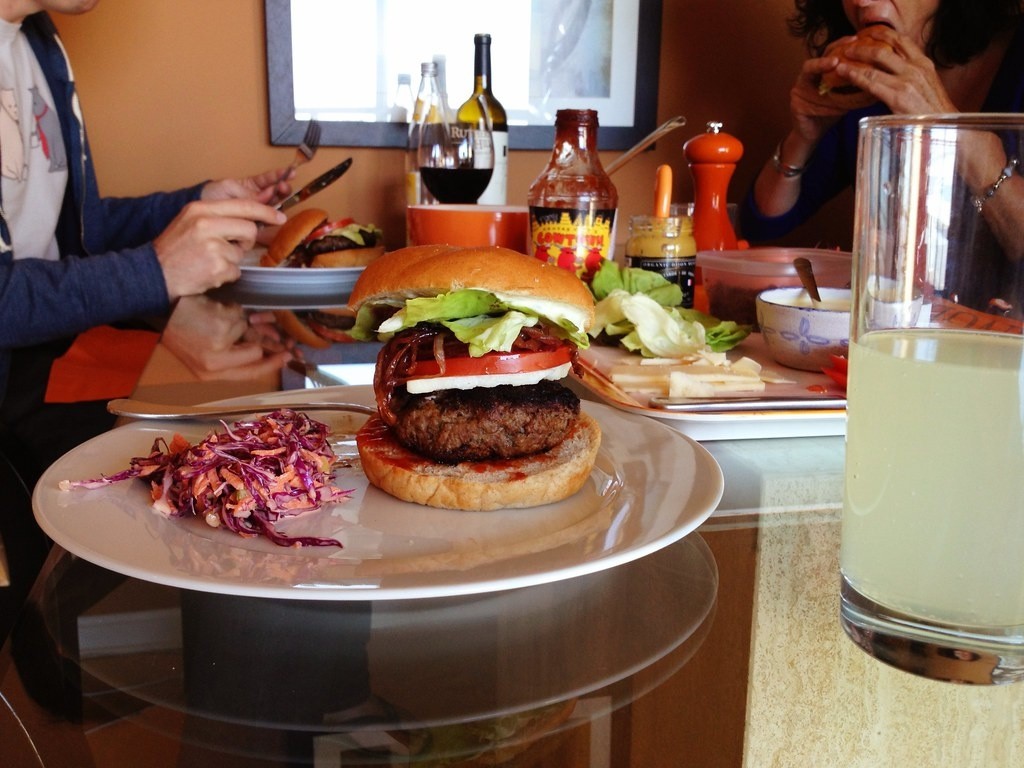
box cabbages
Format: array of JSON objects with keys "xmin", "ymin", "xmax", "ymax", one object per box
[{"xmin": 588, "ymin": 259, "xmax": 754, "ymax": 363}]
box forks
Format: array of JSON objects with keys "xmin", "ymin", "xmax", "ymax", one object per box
[{"xmin": 274, "ymin": 119, "xmax": 321, "ymax": 192}]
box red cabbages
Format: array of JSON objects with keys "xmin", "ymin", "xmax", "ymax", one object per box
[{"xmin": 63, "ymin": 406, "xmax": 358, "ymax": 548}]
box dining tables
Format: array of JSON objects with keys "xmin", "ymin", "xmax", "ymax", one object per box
[{"xmin": 0, "ymin": 297, "xmax": 1024, "ymax": 768}]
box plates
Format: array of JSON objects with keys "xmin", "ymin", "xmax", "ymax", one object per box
[
  {"xmin": 29, "ymin": 383, "xmax": 724, "ymax": 604},
  {"xmin": 38, "ymin": 540, "xmax": 717, "ymax": 733}
]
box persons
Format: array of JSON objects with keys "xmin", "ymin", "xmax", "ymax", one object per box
[
  {"xmin": 0, "ymin": 0, "xmax": 296, "ymax": 348},
  {"xmin": 0, "ymin": 294, "xmax": 433, "ymax": 768},
  {"xmin": 737, "ymin": 0, "xmax": 1024, "ymax": 324}
]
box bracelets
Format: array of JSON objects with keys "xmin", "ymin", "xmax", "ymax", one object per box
[
  {"xmin": 774, "ymin": 136, "xmax": 817, "ymax": 178},
  {"xmin": 968, "ymin": 154, "xmax": 1020, "ymax": 212}
]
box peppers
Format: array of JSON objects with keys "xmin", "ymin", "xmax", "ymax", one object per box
[{"xmin": 821, "ymin": 352, "xmax": 848, "ymax": 390}]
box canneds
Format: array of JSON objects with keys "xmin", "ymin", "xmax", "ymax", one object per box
[{"xmin": 627, "ymin": 214, "xmax": 695, "ymax": 311}]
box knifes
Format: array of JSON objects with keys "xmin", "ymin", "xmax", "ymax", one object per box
[{"xmin": 229, "ymin": 157, "xmax": 352, "ymax": 243}]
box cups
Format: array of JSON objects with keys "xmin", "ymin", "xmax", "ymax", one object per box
[
  {"xmin": 416, "ymin": 96, "xmax": 496, "ymax": 205},
  {"xmin": 835, "ymin": 110, "xmax": 1023, "ymax": 687}
]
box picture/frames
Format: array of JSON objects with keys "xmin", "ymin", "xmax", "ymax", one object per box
[{"xmin": 266, "ymin": 0, "xmax": 664, "ymax": 150}]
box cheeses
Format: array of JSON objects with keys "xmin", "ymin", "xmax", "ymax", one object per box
[{"xmin": 609, "ymin": 357, "xmax": 794, "ymax": 397}]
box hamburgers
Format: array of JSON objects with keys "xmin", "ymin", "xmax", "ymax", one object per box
[
  {"xmin": 817, "ymin": 23, "xmax": 899, "ymax": 110},
  {"xmin": 262, "ymin": 207, "xmax": 387, "ymax": 268},
  {"xmin": 271, "ymin": 305, "xmax": 367, "ymax": 348},
  {"xmin": 348, "ymin": 246, "xmax": 600, "ymax": 510}
]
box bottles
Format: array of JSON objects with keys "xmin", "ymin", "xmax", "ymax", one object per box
[
  {"xmin": 524, "ymin": 110, "xmax": 621, "ymax": 289},
  {"xmin": 457, "ymin": 33, "xmax": 510, "ymax": 207},
  {"xmin": 625, "ymin": 214, "xmax": 698, "ymax": 310},
  {"xmin": 406, "ymin": 61, "xmax": 450, "ymax": 206},
  {"xmin": 389, "ymin": 73, "xmax": 416, "ymax": 124}
]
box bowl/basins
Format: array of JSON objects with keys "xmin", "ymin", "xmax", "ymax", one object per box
[
  {"xmin": 694, "ymin": 246, "xmax": 853, "ymax": 333},
  {"xmin": 404, "ymin": 204, "xmax": 527, "ymax": 257},
  {"xmin": 756, "ymin": 286, "xmax": 854, "ymax": 372},
  {"xmin": 236, "ymin": 246, "xmax": 367, "ymax": 308}
]
